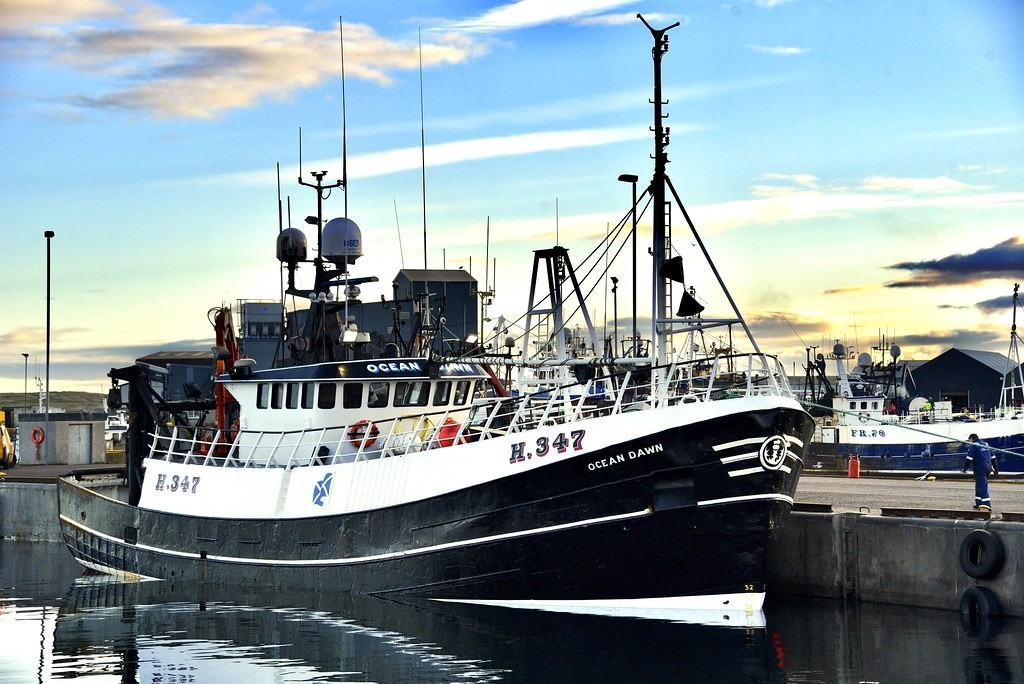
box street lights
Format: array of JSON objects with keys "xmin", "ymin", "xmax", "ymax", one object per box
[{"xmin": 20, "ymin": 352, "xmax": 30, "ymax": 414}]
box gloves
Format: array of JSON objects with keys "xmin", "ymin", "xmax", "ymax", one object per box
[
  {"xmin": 993, "ymin": 469, "xmax": 998, "ymax": 476},
  {"xmin": 962, "ymin": 469, "xmax": 966, "ymax": 473}
]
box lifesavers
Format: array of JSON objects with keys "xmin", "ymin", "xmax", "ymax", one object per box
[
  {"xmin": 32, "ymin": 427, "xmax": 44, "ymax": 444},
  {"xmin": 858, "ymin": 411, "xmax": 870, "ymax": 423},
  {"xmin": 230, "ymin": 418, "xmax": 239, "ymax": 451},
  {"xmin": 960, "ymin": 618, "xmax": 998, "ymax": 640},
  {"xmin": 959, "ymin": 529, "xmax": 1006, "ymax": 580},
  {"xmin": 963, "ymin": 648, "xmax": 1011, "ymax": 684},
  {"xmin": 960, "ymin": 586, "xmax": 998, "ymax": 617},
  {"xmin": 350, "ymin": 419, "xmax": 377, "ymax": 448}
]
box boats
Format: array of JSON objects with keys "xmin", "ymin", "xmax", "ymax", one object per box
[
  {"xmin": 53, "ymin": 11, "xmax": 818, "ymax": 640},
  {"xmin": 792, "ymin": 281, "xmax": 1024, "ymax": 475}
]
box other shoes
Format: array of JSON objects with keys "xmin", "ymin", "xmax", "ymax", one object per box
[{"xmin": 973, "ymin": 505, "xmax": 978, "ymax": 508}]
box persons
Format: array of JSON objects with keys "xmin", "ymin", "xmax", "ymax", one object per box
[
  {"xmin": 890, "ymin": 404, "xmax": 896, "ymax": 414},
  {"xmin": 922, "ymin": 400, "xmax": 932, "ymax": 424},
  {"xmin": 961, "ymin": 433, "xmax": 999, "ymax": 510}
]
